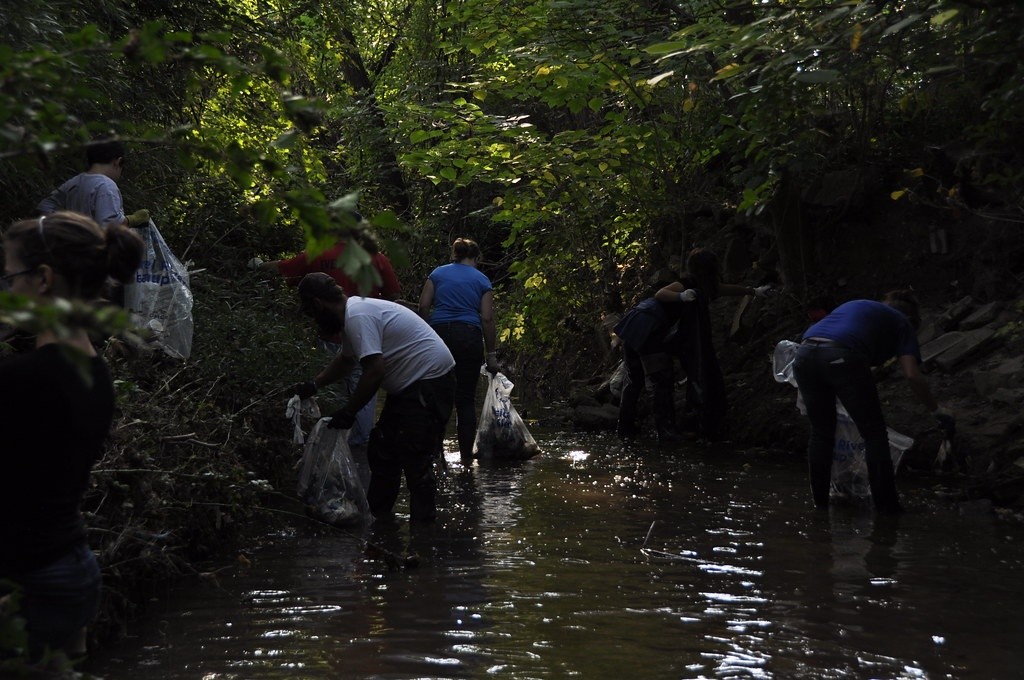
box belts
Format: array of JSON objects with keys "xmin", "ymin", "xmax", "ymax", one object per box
[{"xmin": 802, "ymin": 339, "xmax": 843, "ymax": 348}]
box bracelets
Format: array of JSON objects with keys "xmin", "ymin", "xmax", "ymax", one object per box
[
  {"xmin": 313, "ymin": 380, "xmax": 318, "ymax": 390},
  {"xmin": 672, "ymin": 292, "xmax": 675, "ymax": 300},
  {"xmin": 488, "ymin": 351, "xmax": 497, "ymax": 355}
]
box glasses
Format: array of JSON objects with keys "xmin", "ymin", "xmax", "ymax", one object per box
[{"xmin": 0, "ymin": 269, "xmax": 35, "ymax": 290}]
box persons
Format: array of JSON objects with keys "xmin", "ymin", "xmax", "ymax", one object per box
[
  {"xmin": 247, "ymin": 206, "xmax": 401, "ymax": 514},
  {"xmin": 288, "ymin": 271, "xmax": 455, "ymax": 521},
  {"xmin": 419, "ymin": 237, "xmax": 502, "ymax": 459},
  {"xmin": 35, "ymin": 134, "xmax": 150, "ymax": 308},
  {"xmin": 0, "ymin": 208, "xmax": 147, "ymax": 668},
  {"xmin": 611, "ymin": 247, "xmax": 771, "ymax": 441},
  {"xmin": 792, "ymin": 289, "xmax": 949, "ymax": 510}
]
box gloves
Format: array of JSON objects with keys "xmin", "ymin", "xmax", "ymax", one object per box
[
  {"xmin": 328, "ymin": 408, "xmax": 357, "ymax": 429},
  {"xmin": 127, "ymin": 209, "xmax": 149, "ymax": 228},
  {"xmin": 931, "ymin": 406, "xmax": 956, "ymax": 420},
  {"xmin": 678, "ymin": 288, "xmax": 697, "ymax": 302},
  {"xmin": 752, "ymin": 285, "xmax": 771, "ymax": 299},
  {"xmin": 285, "ymin": 381, "xmax": 319, "ymax": 402}
]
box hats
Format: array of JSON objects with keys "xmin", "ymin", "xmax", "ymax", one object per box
[{"xmin": 294, "ymin": 272, "xmax": 331, "ymax": 312}]
